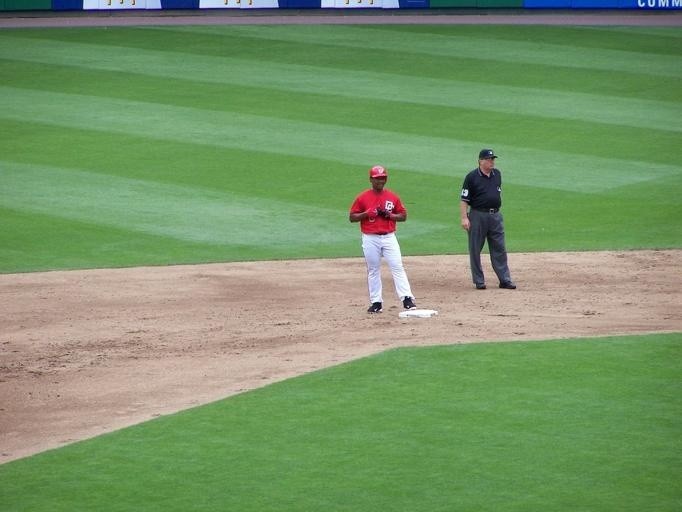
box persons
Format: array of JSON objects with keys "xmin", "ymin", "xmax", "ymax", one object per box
[
  {"xmin": 351, "ymin": 165, "xmax": 419, "ymax": 313},
  {"xmin": 461, "ymin": 149, "xmax": 516, "ymax": 290}
]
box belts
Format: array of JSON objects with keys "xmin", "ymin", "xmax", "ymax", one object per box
[{"xmin": 474, "ymin": 207, "xmax": 498, "ymax": 214}]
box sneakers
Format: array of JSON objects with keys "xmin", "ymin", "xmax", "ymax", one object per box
[
  {"xmin": 368, "ymin": 302, "xmax": 383, "ymax": 313},
  {"xmin": 499, "ymin": 282, "xmax": 516, "ymax": 289},
  {"xmin": 403, "ymin": 296, "xmax": 416, "ymax": 310},
  {"xmin": 476, "ymin": 283, "xmax": 486, "ymax": 289}
]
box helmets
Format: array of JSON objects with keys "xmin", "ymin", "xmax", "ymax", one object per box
[{"xmin": 370, "ymin": 165, "xmax": 387, "ymax": 178}]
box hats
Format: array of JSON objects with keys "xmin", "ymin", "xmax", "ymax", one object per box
[{"xmin": 479, "ymin": 149, "xmax": 497, "ymax": 158}]
